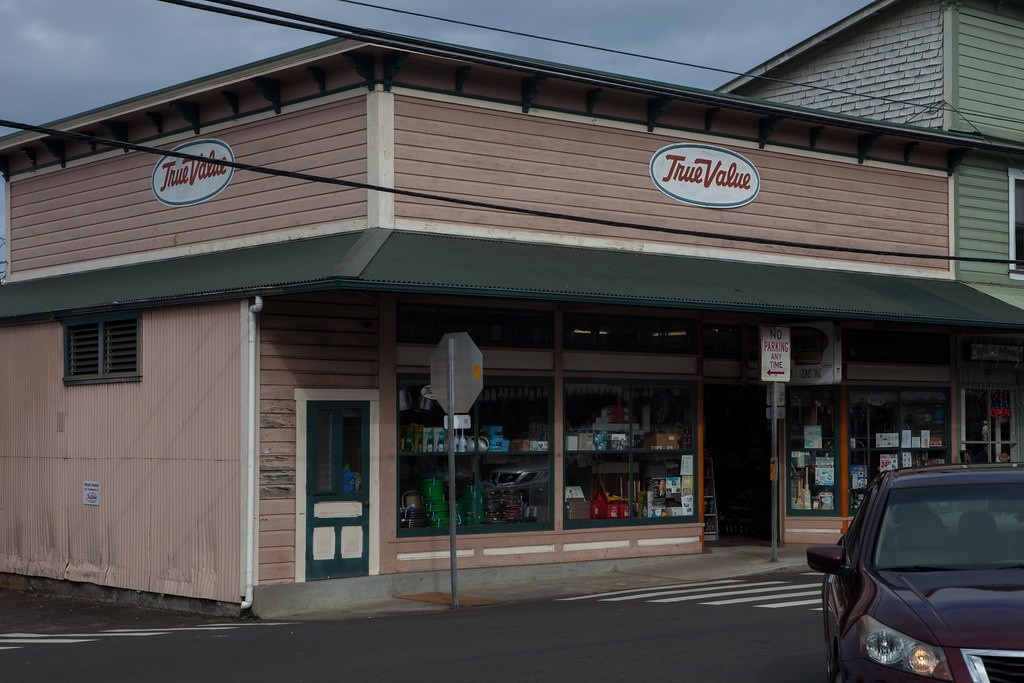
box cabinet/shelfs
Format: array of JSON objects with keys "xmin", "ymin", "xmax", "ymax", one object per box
[
  {"xmin": 395, "ymin": 372, "xmax": 555, "ymax": 539},
  {"xmin": 703, "ymin": 455, "xmax": 719, "ymax": 542},
  {"xmin": 846, "ymin": 385, "xmax": 952, "ymax": 517},
  {"xmin": 563, "ymin": 376, "xmax": 698, "ymax": 530},
  {"xmin": 785, "ymin": 385, "xmax": 842, "ymax": 517}
]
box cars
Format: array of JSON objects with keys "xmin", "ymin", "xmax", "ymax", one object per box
[
  {"xmin": 488, "ymin": 456, "xmax": 644, "ymax": 521},
  {"xmin": 806, "ymin": 458, "xmax": 1024, "ymax": 683}
]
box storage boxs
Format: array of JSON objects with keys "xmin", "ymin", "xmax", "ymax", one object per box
[
  {"xmin": 400, "ymin": 422, "xmax": 446, "ymax": 453},
  {"xmin": 564, "ymin": 406, "xmax": 694, "ymax": 517},
  {"xmin": 849, "ymin": 430, "xmax": 942, "ymax": 490},
  {"xmin": 482, "ymin": 425, "xmax": 550, "ymax": 451},
  {"xmin": 790, "ymin": 425, "xmax": 835, "ymax": 511}
]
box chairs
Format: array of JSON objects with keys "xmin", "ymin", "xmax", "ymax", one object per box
[{"xmin": 889, "ymin": 502, "xmax": 1024, "ymax": 565}]
